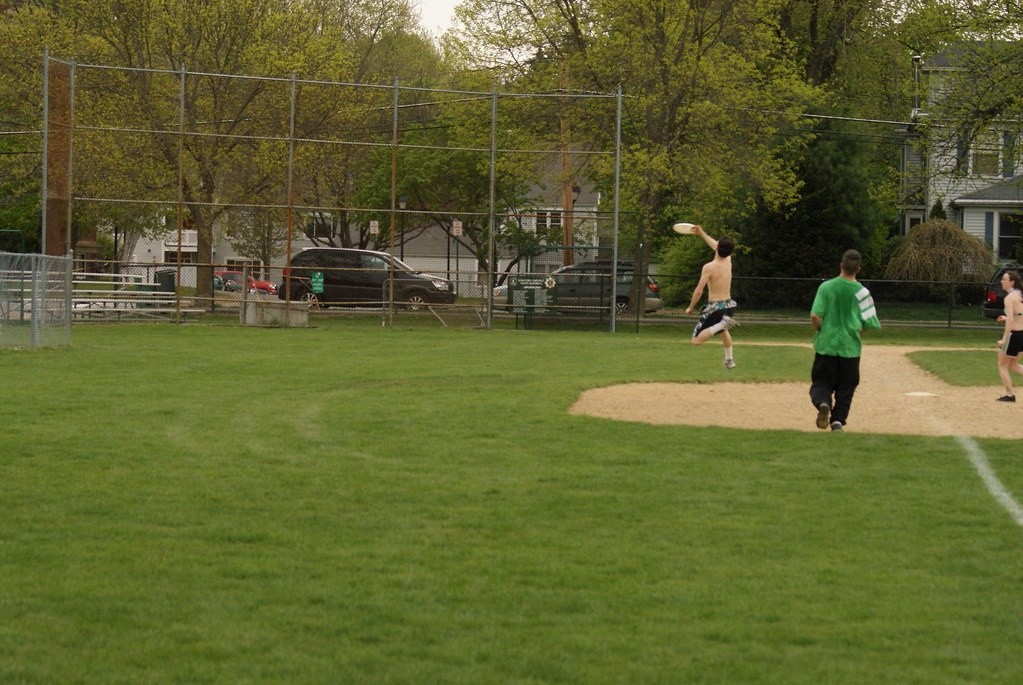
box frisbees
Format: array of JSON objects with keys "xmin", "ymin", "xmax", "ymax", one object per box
[{"xmin": 672, "ymin": 222, "xmax": 696, "ymax": 234}]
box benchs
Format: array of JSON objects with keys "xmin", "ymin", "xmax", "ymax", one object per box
[{"xmin": 0, "ymin": 270, "xmax": 207, "ymax": 328}]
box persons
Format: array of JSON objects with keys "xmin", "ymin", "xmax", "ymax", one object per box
[
  {"xmin": 809, "ymin": 250, "xmax": 881, "ymax": 431},
  {"xmin": 996, "ymin": 271, "xmax": 1023, "ymax": 402},
  {"xmin": 686, "ymin": 225, "xmax": 741, "ymax": 369}
]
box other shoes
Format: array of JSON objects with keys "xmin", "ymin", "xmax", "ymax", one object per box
[
  {"xmin": 721, "ymin": 314, "xmax": 740, "ymax": 328},
  {"xmin": 723, "ymin": 359, "xmax": 736, "ymax": 369},
  {"xmin": 831, "ymin": 421, "xmax": 842, "ymax": 432},
  {"xmin": 816, "ymin": 402, "xmax": 830, "ymax": 430}
]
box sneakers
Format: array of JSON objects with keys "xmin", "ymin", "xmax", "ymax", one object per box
[{"xmin": 996, "ymin": 395, "xmax": 1015, "ymax": 402}]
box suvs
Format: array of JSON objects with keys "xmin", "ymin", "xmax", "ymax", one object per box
[
  {"xmin": 492, "ymin": 259, "xmax": 663, "ymax": 317},
  {"xmin": 982, "ymin": 265, "xmax": 1023, "ymax": 318},
  {"xmin": 279, "ymin": 247, "xmax": 457, "ymax": 313},
  {"xmin": 212, "ymin": 271, "xmax": 278, "ymax": 296}
]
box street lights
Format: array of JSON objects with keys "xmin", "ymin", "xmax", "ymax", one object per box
[{"xmin": 398, "ymin": 194, "xmax": 408, "ymax": 262}]
